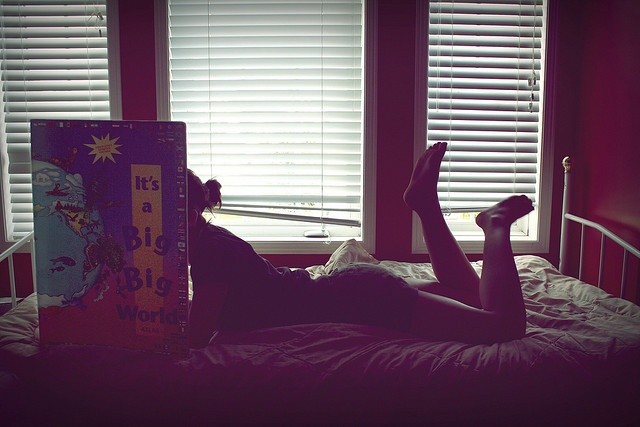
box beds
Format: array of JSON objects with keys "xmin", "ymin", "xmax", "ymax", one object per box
[{"xmin": 0, "ymin": 155, "xmax": 639, "ymax": 426}]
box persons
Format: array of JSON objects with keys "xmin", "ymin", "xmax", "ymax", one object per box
[{"xmin": 187, "ymin": 141, "xmax": 535, "ymax": 348}]
